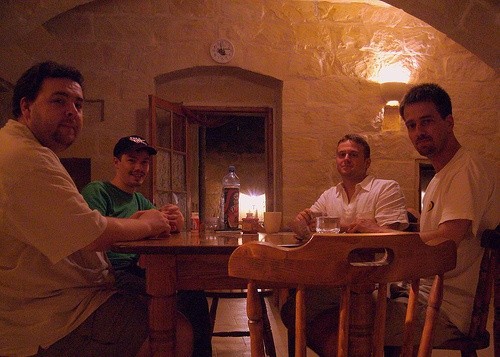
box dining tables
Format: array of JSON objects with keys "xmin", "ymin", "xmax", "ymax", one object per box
[{"xmin": 113, "ymin": 230, "xmax": 385, "ymax": 357}]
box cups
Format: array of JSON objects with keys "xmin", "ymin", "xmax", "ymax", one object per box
[
  {"xmin": 242, "ymin": 218, "xmax": 259, "ymax": 234},
  {"xmin": 316, "ymin": 217, "xmax": 340, "ymax": 234},
  {"xmin": 205, "ymin": 217, "xmax": 218, "ymax": 232},
  {"xmin": 264, "ymin": 212, "xmax": 282, "ymax": 234}
]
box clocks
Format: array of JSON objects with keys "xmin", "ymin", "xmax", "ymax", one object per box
[{"xmin": 211, "ymin": 39, "xmax": 234, "ymax": 63}]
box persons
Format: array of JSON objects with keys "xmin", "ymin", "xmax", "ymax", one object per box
[
  {"xmin": 307, "ymin": 84, "xmax": 500, "ymax": 357},
  {"xmin": 0, "ymin": 60, "xmax": 193, "ymax": 357},
  {"xmin": 281, "ymin": 135, "xmax": 408, "ymax": 294},
  {"xmin": 82, "ymin": 135, "xmax": 213, "ymax": 356}
]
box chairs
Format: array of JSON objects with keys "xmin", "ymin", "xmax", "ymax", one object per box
[
  {"xmin": 204, "ymin": 289, "xmax": 276, "ymax": 357},
  {"xmin": 229, "ymin": 234, "xmax": 457, "ymax": 357},
  {"xmin": 390, "ymin": 229, "xmax": 500, "ymax": 357}
]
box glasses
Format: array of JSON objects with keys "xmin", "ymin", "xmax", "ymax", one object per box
[{"xmin": 336, "ymin": 151, "xmax": 367, "ymax": 158}]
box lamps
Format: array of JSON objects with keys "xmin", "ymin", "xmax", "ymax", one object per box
[{"xmin": 380, "ymin": 82, "xmax": 408, "ymax": 106}]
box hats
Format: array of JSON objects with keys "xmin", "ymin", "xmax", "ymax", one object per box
[{"xmin": 114, "ymin": 135, "xmax": 157, "ymax": 157}]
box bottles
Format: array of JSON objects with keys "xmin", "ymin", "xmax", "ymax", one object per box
[
  {"xmin": 348, "ymin": 290, "xmax": 374, "ymax": 357},
  {"xmin": 191, "ymin": 213, "xmax": 199, "ymax": 233},
  {"xmin": 221, "ymin": 166, "xmax": 241, "ymax": 231}
]
